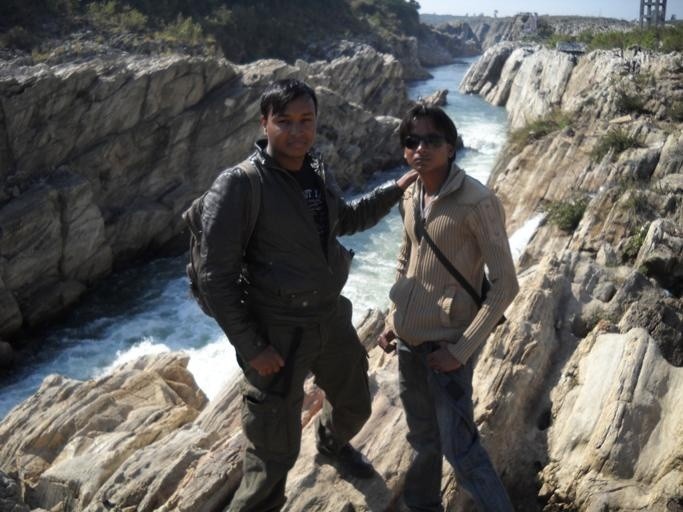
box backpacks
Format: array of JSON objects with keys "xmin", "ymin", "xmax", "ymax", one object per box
[{"xmin": 181, "ymin": 159, "xmax": 263, "ymax": 318}]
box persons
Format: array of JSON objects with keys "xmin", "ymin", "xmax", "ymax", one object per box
[
  {"xmin": 193, "ymin": 79, "xmax": 421, "ymax": 511},
  {"xmin": 374, "ymin": 105, "xmax": 520, "ymax": 511}
]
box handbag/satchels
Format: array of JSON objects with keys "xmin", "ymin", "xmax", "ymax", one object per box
[{"xmin": 480, "ymin": 271, "xmax": 507, "ymax": 330}]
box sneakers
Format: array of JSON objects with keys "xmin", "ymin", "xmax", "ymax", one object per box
[{"xmin": 314, "ymin": 438, "xmax": 374, "ymax": 479}]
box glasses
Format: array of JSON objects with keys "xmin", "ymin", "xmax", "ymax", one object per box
[{"xmin": 402, "ymin": 132, "xmax": 451, "ymax": 149}]
